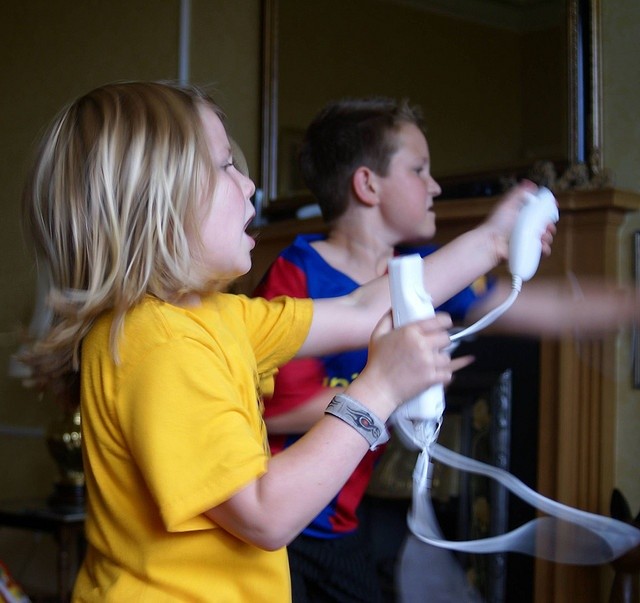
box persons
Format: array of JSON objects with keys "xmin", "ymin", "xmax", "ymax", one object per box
[
  {"xmin": 252, "ymin": 98, "xmax": 640, "ymax": 602},
  {"xmin": 12, "ymin": 81, "xmax": 558, "ymax": 603}
]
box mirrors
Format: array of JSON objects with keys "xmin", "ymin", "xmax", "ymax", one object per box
[{"xmin": 258, "ymin": 2, "xmax": 603, "ymax": 218}]
